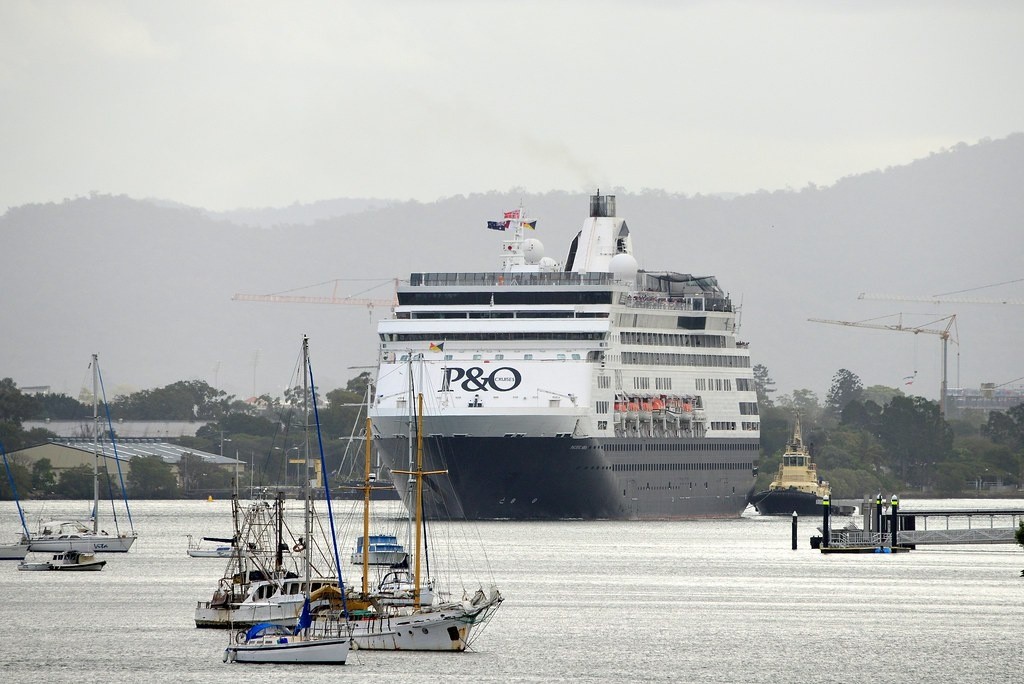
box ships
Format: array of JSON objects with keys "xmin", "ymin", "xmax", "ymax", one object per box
[{"xmin": 366, "ymin": 190, "xmax": 762, "ymax": 524}]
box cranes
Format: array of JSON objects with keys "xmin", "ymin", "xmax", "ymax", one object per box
[
  {"xmin": 858, "ymin": 277, "xmax": 1024, "ymax": 306},
  {"xmin": 806, "ymin": 313, "xmax": 960, "ymax": 424},
  {"xmin": 232, "ymin": 274, "xmax": 408, "ymax": 307}
]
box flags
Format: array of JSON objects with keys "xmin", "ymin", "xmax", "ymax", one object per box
[
  {"xmin": 429, "ymin": 341, "xmax": 446, "ymax": 353},
  {"xmin": 487, "ymin": 209, "xmax": 537, "ymax": 232}
]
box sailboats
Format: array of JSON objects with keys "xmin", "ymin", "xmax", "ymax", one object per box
[
  {"xmin": 26, "ymin": 354, "xmax": 138, "ymax": 553},
  {"xmin": 192, "ymin": 329, "xmax": 503, "ymax": 665},
  {"xmin": 332, "ymin": 377, "xmax": 403, "ymax": 502},
  {"xmin": 0, "ymin": 433, "xmax": 32, "ymax": 560}
]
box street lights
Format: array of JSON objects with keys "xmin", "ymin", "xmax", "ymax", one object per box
[{"xmin": 274, "ymin": 446, "xmax": 298, "ymax": 486}]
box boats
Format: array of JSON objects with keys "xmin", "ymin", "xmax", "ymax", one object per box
[
  {"xmin": 349, "ymin": 534, "xmax": 413, "ymax": 570},
  {"xmin": 639, "ymin": 401, "xmax": 651, "ymax": 421},
  {"xmin": 682, "ymin": 402, "xmax": 695, "ymax": 421},
  {"xmin": 17, "ymin": 549, "xmax": 108, "ymax": 573},
  {"xmin": 615, "ymin": 403, "xmax": 627, "ymax": 420},
  {"xmin": 626, "ymin": 402, "xmax": 638, "ymax": 421},
  {"xmin": 666, "ymin": 399, "xmax": 682, "ymax": 422},
  {"xmin": 748, "ymin": 411, "xmax": 831, "ymax": 517},
  {"xmin": 652, "ymin": 398, "xmax": 665, "ymax": 421},
  {"xmin": 187, "ymin": 534, "xmax": 256, "ymax": 558}
]
